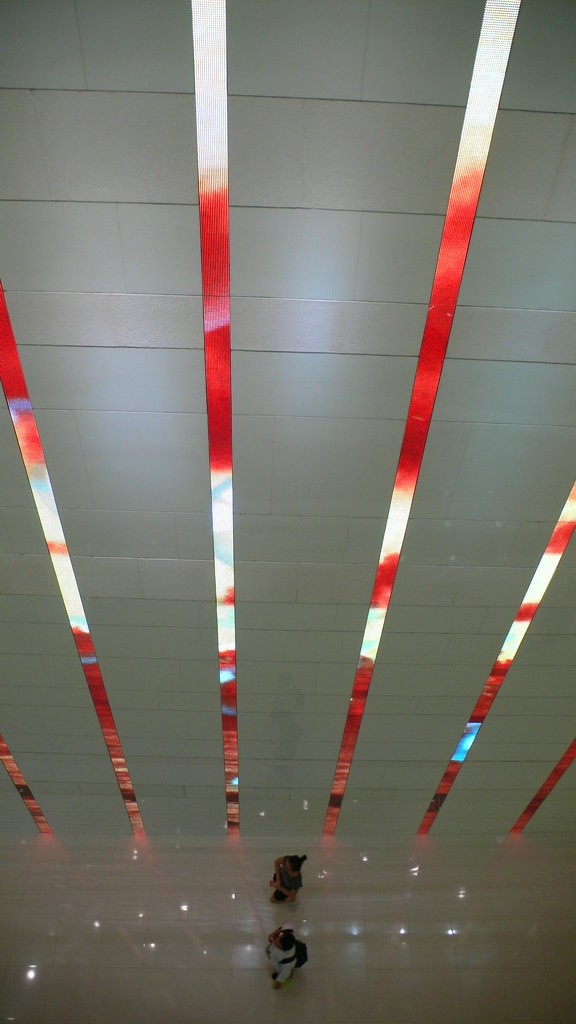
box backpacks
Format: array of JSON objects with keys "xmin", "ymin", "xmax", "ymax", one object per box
[{"xmin": 278, "ymin": 929, "xmax": 308, "ymax": 968}]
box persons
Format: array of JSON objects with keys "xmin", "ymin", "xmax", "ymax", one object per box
[
  {"xmin": 266, "ymin": 929, "xmax": 297, "ymax": 990},
  {"xmin": 269, "ymin": 854, "xmax": 307, "ymax": 904}
]
